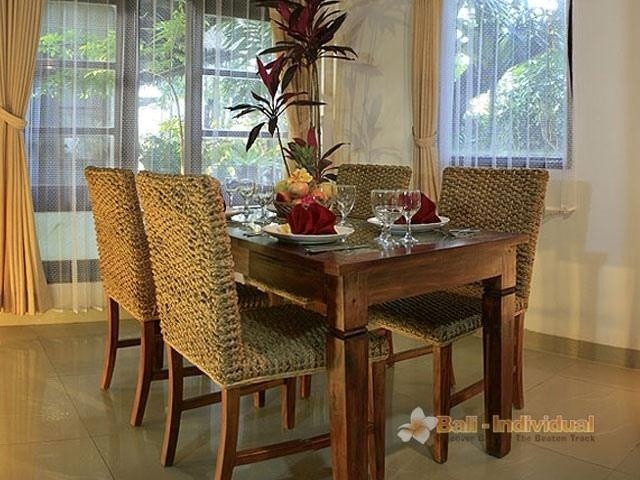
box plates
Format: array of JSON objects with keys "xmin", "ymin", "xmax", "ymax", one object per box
[{"xmin": 366, "ymin": 215, "xmax": 451, "ymax": 233}]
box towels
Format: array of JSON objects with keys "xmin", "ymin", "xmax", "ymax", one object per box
[
  {"xmin": 288, "ymin": 202, "xmax": 337, "ymax": 234},
  {"xmin": 394, "ymin": 192, "xmax": 441, "ymax": 224}
]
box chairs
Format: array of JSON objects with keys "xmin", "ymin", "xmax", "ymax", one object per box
[
  {"xmin": 366, "ymin": 167, "xmax": 549, "ymax": 464},
  {"xmin": 85, "ymin": 166, "xmax": 283, "ymax": 427},
  {"xmin": 135, "ymin": 171, "xmax": 391, "ymax": 480},
  {"xmin": 301, "ymin": 163, "xmax": 455, "ymax": 399}
]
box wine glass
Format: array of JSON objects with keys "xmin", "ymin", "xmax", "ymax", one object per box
[{"xmin": 224, "ymin": 175, "xmax": 422, "ymax": 248}]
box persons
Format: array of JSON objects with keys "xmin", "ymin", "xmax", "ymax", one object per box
[{"xmin": 202, "ymin": 151, "xmax": 235, "ymax": 176}]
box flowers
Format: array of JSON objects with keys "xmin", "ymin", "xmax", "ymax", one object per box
[
  {"xmin": 224, "ymin": 0, "xmax": 357, "ymax": 183},
  {"xmin": 397, "ymin": 406, "xmax": 438, "ymax": 444}
]
